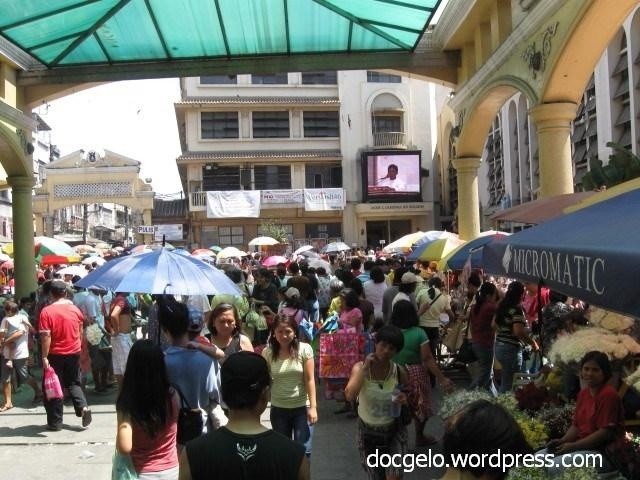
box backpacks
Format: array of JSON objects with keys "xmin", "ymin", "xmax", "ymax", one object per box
[{"xmin": 111, "ymin": 453, "xmax": 137, "ymax": 480}]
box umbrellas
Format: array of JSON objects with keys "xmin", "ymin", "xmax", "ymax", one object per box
[{"xmin": 382, "ymin": 228, "xmax": 514, "ymax": 273}]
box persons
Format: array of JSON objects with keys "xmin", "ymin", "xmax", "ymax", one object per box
[
  {"xmin": 1, "ymin": 238, "xmax": 639, "ymax": 479},
  {"xmin": 376, "ymin": 164, "xmax": 405, "ymax": 191}
]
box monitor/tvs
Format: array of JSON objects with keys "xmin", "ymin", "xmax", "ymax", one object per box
[{"xmin": 361, "ymin": 150, "xmax": 422, "ymax": 204}]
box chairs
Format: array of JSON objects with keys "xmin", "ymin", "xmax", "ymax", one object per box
[{"xmin": 512, "ymin": 368, "xmax": 545, "ymax": 391}]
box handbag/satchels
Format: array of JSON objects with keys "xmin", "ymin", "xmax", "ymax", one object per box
[
  {"xmin": 457, "ymin": 337, "xmax": 478, "ymax": 364},
  {"xmin": 398, "ymin": 404, "xmax": 414, "ymax": 427}
]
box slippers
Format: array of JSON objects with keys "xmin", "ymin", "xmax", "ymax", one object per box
[
  {"xmin": 32, "ymin": 390, "xmax": 44, "ymax": 403},
  {"xmin": 0, "ymin": 402, "xmax": 14, "ymax": 412}
]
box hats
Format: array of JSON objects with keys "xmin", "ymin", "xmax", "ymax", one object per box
[
  {"xmin": 401, "ymin": 272, "xmax": 422, "ymax": 284},
  {"xmin": 220, "ymin": 350, "xmax": 275, "ymax": 390},
  {"xmin": 284, "ymin": 287, "xmax": 301, "ymax": 300}
]
